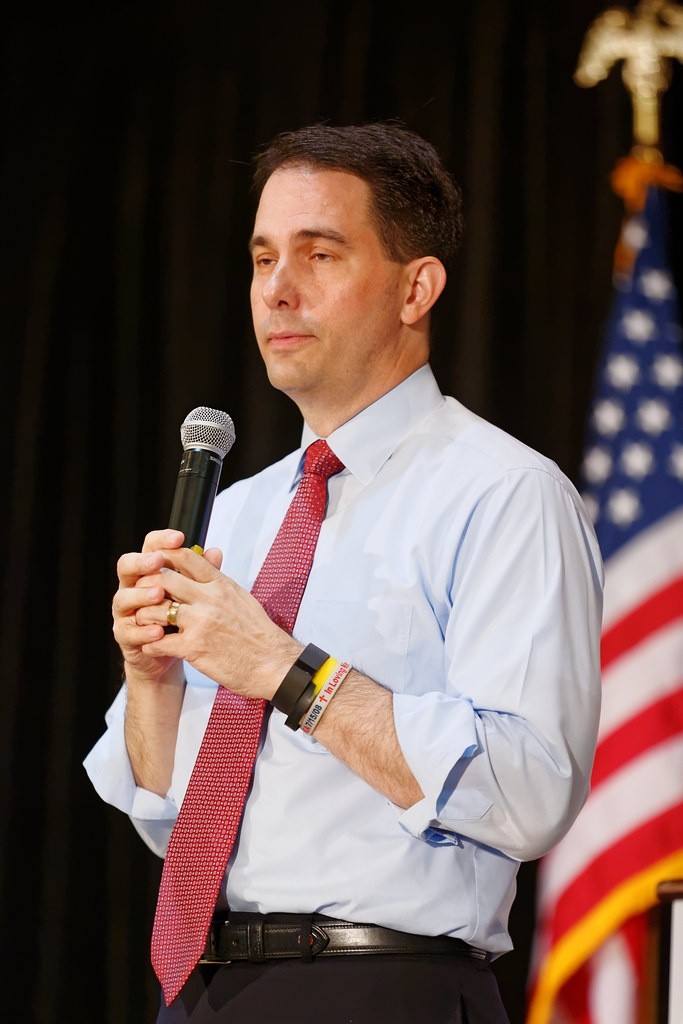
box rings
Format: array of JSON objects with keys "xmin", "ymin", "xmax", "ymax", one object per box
[{"xmin": 167, "ymin": 602, "xmax": 181, "ymax": 626}]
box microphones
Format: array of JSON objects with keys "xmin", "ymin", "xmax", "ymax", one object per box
[{"xmin": 159, "ymin": 407, "xmax": 237, "ymax": 638}]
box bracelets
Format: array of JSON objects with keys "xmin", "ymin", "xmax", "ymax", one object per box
[{"xmin": 269, "ymin": 642, "xmax": 353, "ymax": 735}]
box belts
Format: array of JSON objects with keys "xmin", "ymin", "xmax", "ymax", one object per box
[{"xmin": 196, "ymin": 909, "xmax": 471, "ymax": 964}]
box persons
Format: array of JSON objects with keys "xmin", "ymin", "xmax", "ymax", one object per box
[{"xmin": 81, "ymin": 129, "xmax": 605, "ymax": 1024}]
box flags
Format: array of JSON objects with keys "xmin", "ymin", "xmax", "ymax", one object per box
[{"xmin": 527, "ymin": 155, "xmax": 683, "ymax": 1024}]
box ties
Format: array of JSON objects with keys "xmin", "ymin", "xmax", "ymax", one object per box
[{"xmin": 151, "ymin": 439, "xmax": 347, "ymax": 1007}]
